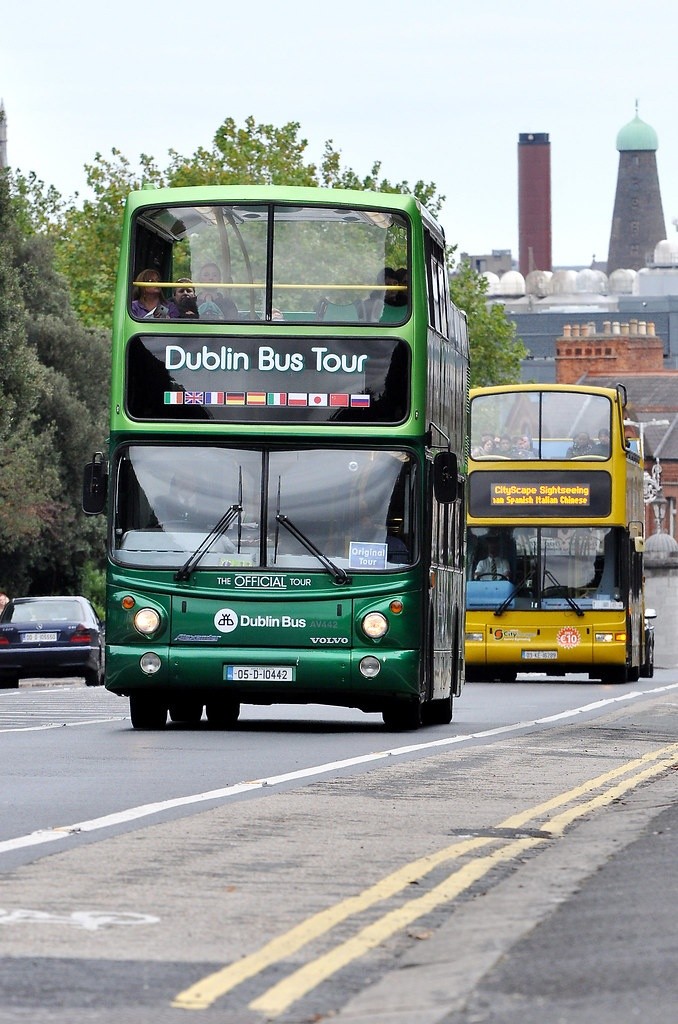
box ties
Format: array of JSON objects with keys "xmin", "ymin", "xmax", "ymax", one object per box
[{"xmin": 491, "ymin": 558, "xmax": 498, "ymax": 581}]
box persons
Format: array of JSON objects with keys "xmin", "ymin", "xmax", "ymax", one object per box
[
  {"xmin": 395, "ymin": 268, "xmax": 408, "ymax": 294},
  {"xmin": 347, "ymin": 509, "xmax": 407, "ymax": 564},
  {"xmin": 472, "ymin": 434, "xmax": 535, "ymax": 457},
  {"xmin": 0, "ymin": 594, "xmax": 10, "ymax": 614},
  {"xmin": 169, "ymin": 278, "xmax": 195, "ymax": 301},
  {"xmin": 364, "ymin": 267, "xmax": 407, "ymax": 320},
  {"xmin": 181, "ymin": 264, "xmax": 238, "ymax": 318},
  {"xmin": 144, "ymin": 469, "xmax": 213, "ymax": 532},
  {"xmin": 130, "ymin": 270, "xmax": 181, "ymax": 320},
  {"xmin": 563, "ymin": 429, "xmax": 630, "ymax": 457},
  {"xmin": 474, "ymin": 541, "xmax": 510, "ymax": 580}
]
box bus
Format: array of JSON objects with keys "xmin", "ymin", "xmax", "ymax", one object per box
[
  {"xmin": 462, "ymin": 384, "xmax": 645, "ymax": 684},
  {"xmin": 80, "ymin": 184, "xmax": 470, "ymax": 731}
]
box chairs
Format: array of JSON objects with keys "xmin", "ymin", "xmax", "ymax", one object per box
[{"xmin": 316, "ymin": 299, "xmax": 409, "ymax": 326}]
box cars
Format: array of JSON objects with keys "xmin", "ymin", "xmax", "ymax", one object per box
[{"xmin": 1, "ymin": 597, "xmax": 106, "ymax": 688}]
